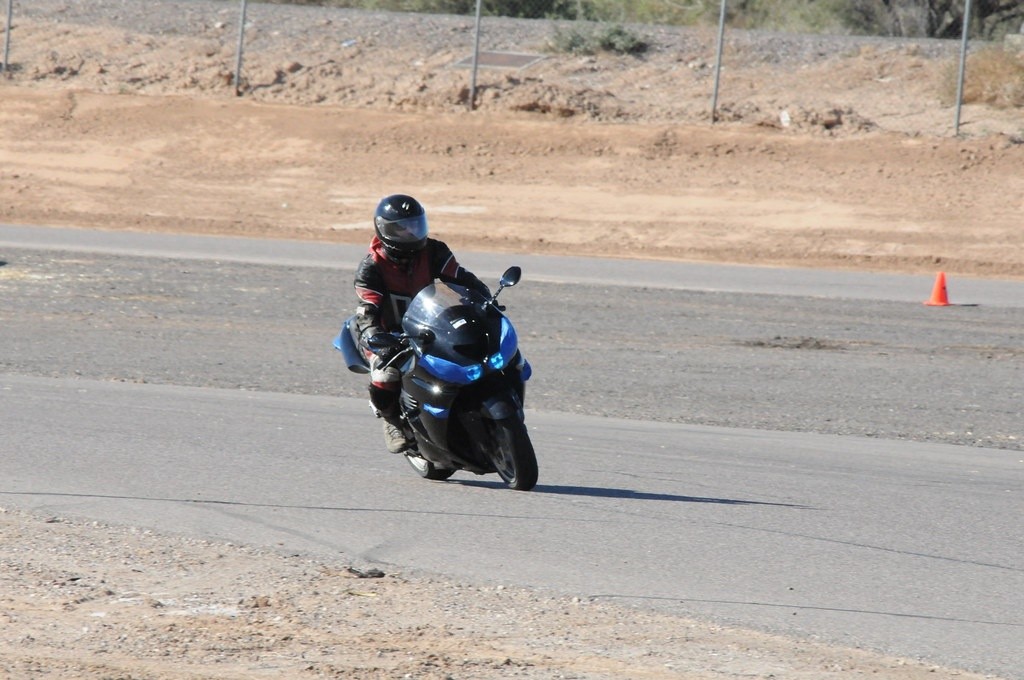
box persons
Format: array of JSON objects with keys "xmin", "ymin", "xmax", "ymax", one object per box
[{"xmin": 354, "ymin": 194, "xmax": 499, "ymax": 454}]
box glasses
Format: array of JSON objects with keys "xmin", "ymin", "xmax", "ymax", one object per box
[{"xmin": 394, "ymin": 221, "xmax": 419, "ymax": 230}]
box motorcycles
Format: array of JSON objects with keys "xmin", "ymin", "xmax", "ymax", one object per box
[{"xmin": 332, "ymin": 267, "xmax": 538, "ymax": 490}]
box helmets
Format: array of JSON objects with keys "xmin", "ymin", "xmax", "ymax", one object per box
[{"xmin": 374, "ymin": 194, "xmax": 428, "ymax": 264}]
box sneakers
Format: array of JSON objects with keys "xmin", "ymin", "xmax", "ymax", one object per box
[{"xmin": 383, "ymin": 415, "xmax": 408, "ymax": 453}]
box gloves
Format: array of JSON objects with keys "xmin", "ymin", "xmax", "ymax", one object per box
[{"xmin": 359, "ymin": 325, "xmax": 407, "ymax": 369}]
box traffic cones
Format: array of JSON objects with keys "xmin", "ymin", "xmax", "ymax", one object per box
[{"xmin": 922, "ymin": 272, "xmax": 949, "ymax": 306}]
